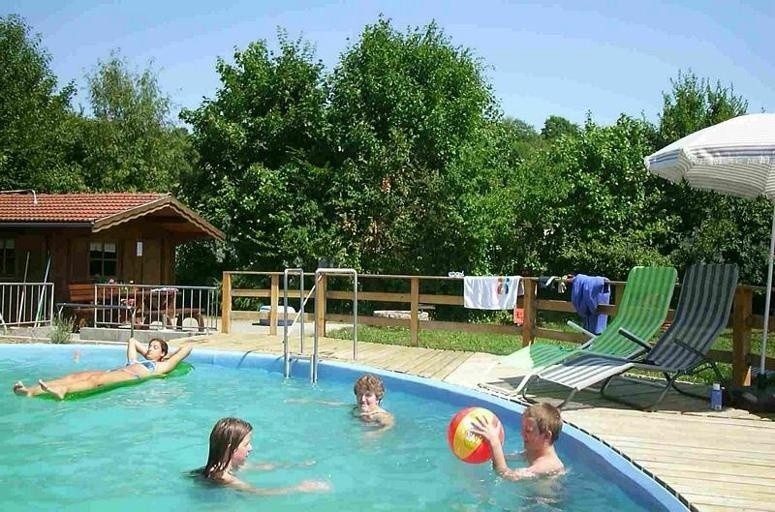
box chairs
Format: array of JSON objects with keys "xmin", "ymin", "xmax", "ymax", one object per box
[
  {"xmin": 521, "ymin": 263, "xmax": 738, "ymax": 412},
  {"xmin": 477, "ymin": 266, "xmax": 677, "ymax": 408}
]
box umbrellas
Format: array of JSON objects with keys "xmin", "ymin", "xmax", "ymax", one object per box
[{"xmin": 644, "ymin": 107, "xmax": 775, "ymax": 374}]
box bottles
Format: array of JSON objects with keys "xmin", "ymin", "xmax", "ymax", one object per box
[
  {"xmin": 711, "ymin": 382, "xmax": 722, "ymax": 412},
  {"xmin": 448, "ymin": 272, "xmax": 464, "ymax": 277}
]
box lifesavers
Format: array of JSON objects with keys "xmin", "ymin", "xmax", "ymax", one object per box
[
  {"xmin": 143, "ymin": 313, "xmax": 173, "ymax": 330},
  {"xmin": 177, "ymin": 311, "xmax": 204, "ymax": 332}
]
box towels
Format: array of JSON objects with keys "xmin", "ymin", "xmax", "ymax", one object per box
[{"xmin": 464, "ymin": 275, "xmax": 524, "ymax": 311}]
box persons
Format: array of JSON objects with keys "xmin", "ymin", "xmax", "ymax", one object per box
[
  {"xmin": 283, "ymin": 375, "xmax": 395, "ymax": 437},
  {"xmin": 13, "ymin": 338, "xmax": 193, "ymax": 400},
  {"xmin": 186, "ymin": 417, "xmax": 331, "ymax": 495},
  {"xmin": 469, "ymin": 402, "xmax": 567, "ymax": 512}
]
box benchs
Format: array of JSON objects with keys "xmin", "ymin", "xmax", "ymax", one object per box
[
  {"xmin": 141, "ymin": 308, "xmax": 206, "ymax": 332},
  {"xmin": 67, "ymin": 281, "xmax": 119, "ymax": 325}
]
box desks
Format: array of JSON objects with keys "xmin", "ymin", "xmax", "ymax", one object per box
[{"xmin": 109, "ymin": 291, "xmax": 181, "ymax": 329}]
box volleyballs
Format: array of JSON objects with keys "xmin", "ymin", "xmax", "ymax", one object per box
[{"xmin": 448, "ymin": 407, "xmax": 504, "ymax": 463}]
